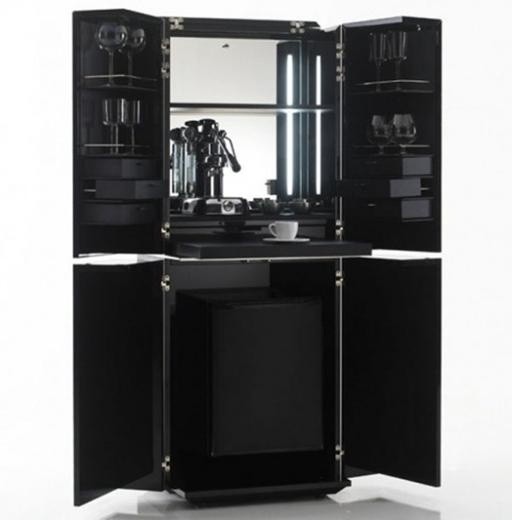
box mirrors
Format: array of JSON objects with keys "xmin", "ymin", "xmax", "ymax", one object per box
[{"xmin": 169, "ymin": 16, "xmax": 333, "ymax": 232}]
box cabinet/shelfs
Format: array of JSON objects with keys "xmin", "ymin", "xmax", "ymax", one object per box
[
  {"xmin": 73, "ymin": 9, "xmax": 170, "ymax": 257},
  {"xmin": 74, "ymin": 259, "xmax": 162, "ymax": 505},
  {"xmin": 341, "ymin": 257, "xmax": 439, "ymax": 486},
  {"xmin": 332, "ymin": 16, "xmax": 440, "ymax": 252}
]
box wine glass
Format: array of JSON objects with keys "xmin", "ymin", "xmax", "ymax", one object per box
[
  {"xmin": 92, "ymin": 20, "xmax": 148, "ymax": 87},
  {"xmin": 366, "ymin": 110, "xmax": 418, "ymax": 155},
  {"xmin": 101, "ymin": 99, "xmax": 145, "ymax": 159},
  {"xmin": 371, "ymin": 31, "xmax": 409, "ymax": 92}
]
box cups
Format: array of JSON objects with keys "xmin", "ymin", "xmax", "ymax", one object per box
[{"xmin": 268, "ymin": 219, "xmax": 300, "ymax": 239}]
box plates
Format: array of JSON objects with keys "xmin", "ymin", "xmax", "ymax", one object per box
[{"xmin": 263, "ymin": 237, "xmax": 310, "ymax": 243}]
